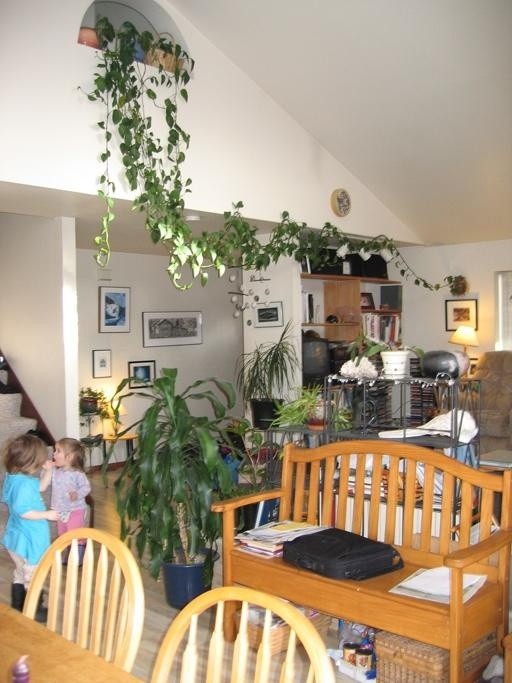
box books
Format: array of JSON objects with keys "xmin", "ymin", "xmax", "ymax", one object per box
[
  {"xmin": 231, "ymin": 519, "xmax": 327, "ymax": 562},
  {"xmin": 362, "ymin": 313, "xmax": 401, "ymax": 345},
  {"xmin": 302, "ymin": 292, "xmax": 309, "ymax": 323},
  {"xmin": 308, "ymin": 293, "xmax": 314, "ymax": 323},
  {"xmin": 324, "ymin": 454, "xmax": 472, "ymax": 511}
]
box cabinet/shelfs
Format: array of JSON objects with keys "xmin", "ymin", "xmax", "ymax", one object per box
[
  {"xmin": 79, "ymin": 411, "xmax": 107, "ymax": 473},
  {"xmin": 101, "ymin": 433, "xmax": 138, "ymax": 469},
  {"xmin": 321, "ymin": 375, "xmax": 483, "ymax": 539},
  {"xmin": 299, "ymin": 271, "xmax": 405, "ymax": 390}
]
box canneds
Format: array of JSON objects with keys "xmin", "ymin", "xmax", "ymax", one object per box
[{"xmin": 343, "ymin": 642, "xmax": 373, "ymax": 673}]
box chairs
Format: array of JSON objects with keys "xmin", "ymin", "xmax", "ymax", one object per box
[
  {"xmin": 147, "ymin": 584, "xmax": 337, "ymax": 682},
  {"xmin": 17, "ymin": 523, "xmax": 146, "ymax": 672}
]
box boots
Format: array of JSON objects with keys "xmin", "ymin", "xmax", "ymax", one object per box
[
  {"xmin": 11, "ymin": 583, "xmax": 27, "ymax": 611},
  {"xmin": 60, "ymin": 545, "xmax": 71, "ymax": 566},
  {"xmin": 76, "ymin": 543, "xmax": 85, "ymax": 567},
  {"xmin": 34, "ymin": 589, "xmax": 47, "ymax": 622}
]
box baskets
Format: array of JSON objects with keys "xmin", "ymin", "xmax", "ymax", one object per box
[
  {"xmin": 232, "ymin": 602, "xmax": 330, "ymax": 658},
  {"xmin": 146, "ymin": 46, "xmax": 185, "ymax": 74},
  {"xmin": 374, "ymin": 630, "xmax": 496, "ymax": 683}
]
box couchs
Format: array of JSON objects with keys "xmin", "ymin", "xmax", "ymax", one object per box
[{"xmin": 433, "ymin": 349, "xmax": 512, "ymax": 464}]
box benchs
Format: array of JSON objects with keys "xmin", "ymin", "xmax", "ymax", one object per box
[{"xmin": 210, "ymin": 438, "xmax": 512, "ymax": 683}]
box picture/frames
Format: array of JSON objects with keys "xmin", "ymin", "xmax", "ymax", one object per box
[
  {"xmin": 125, "ymin": 357, "xmax": 159, "ymax": 389},
  {"xmin": 254, "ymin": 299, "xmax": 286, "ymax": 329},
  {"xmin": 89, "ymin": 346, "xmax": 115, "ymax": 380},
  {"xmin": 96, "ymin": 282, "xmax": 132, "ymax": 335},
  {"xmin": 443, "ymin": 298, "xmax": 478, "ymax": 329},
  {"xmin": 141, "ymin": 308, "xmax": 205, "ymax": 346},
  {"xmin": 360, "ymin": 292, "xmax": 375, "ymax": 309}
]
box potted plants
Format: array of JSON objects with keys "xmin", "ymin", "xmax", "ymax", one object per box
[
  {"xmin": 78, "ymin": 386, "xmax": 110, "ymax": 420},
  {"xmin": 114, "ymin": 364, "xmax": 262, "ymax": 611},
  {"xmin": 234, "ymin": 317, "xmax": 300, "ymax": 429},
  {"xmin": 239, "ymin": 439, "xmax": 282, "ymax": 531},
  {"xmin": 345, "ymin": 329, "xmax": 425, "ymax": 377},
  {"xmin": 76, "ymin": 14, "xmax": 474, "ymax": 296}
]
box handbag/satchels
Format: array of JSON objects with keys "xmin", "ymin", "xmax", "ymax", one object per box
[{"xmin": 284, "ymin": 528, "xmax": 396, "ymax": 581}]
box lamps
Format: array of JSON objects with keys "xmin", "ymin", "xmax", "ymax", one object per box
[
  {"xmin": 448, "ymin": 324, "xmax": 480, "ymax": 359},
  {"xmin": 103, "ymin": 400, "xmax": 130, "ymax": 437}
]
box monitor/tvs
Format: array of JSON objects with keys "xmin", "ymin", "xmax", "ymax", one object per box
[{"xmin": 303, "ymin": 335, "xmax": 329, "ymax": 376}]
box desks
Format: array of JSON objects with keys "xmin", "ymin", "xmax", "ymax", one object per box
[{"xmin": 0, "ymin": 597, "xmax": 148, "ymax": 682}]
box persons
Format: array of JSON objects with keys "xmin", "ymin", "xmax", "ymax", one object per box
[
  {"xmin": 0, "ymin": 433, "xmax": 64, "ymax": 623},
  {"xmin": 39, "ymin": 436, "xmax": 94, "ymax": 568}
]
